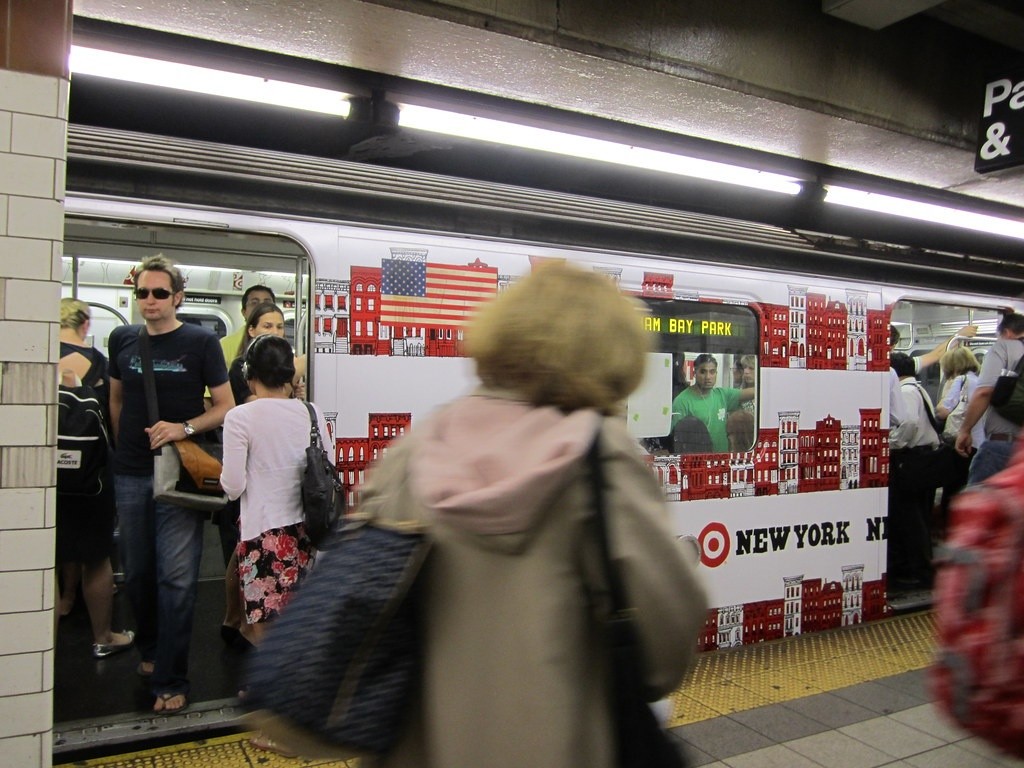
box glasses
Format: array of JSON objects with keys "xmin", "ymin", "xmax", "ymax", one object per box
[
  {"xmin": 245, "ymin": 299, "xmax": 275, "ymax": 306},
  {"xmin": 134, "ymin": 287, "xmax": 177, "ymax": 299}
]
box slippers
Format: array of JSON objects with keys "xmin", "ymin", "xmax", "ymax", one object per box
[
  {"xmin": 153, "ymin": 692, "xmax": 187, "ymax": 713},
  {"xmin": 250, "ymin": 735, "xmax": 298, "ymax": 757},
  {"xmin": 136, "ymin": 662, "xmax": 159, "ymax": 673}
]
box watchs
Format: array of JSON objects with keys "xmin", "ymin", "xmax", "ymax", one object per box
[{"xmin": 182, "ymin": 421, "xmax": 195, "ymax": 438}]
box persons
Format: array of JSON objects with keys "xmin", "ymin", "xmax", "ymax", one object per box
[
  {"xmin": 56, "ymin": 254, "xmax": 336, "ymax": 758},
  {"xmin": 359, "ymin": 262, "xmax": 711, "ymax": 768},
  {"xmin": 639, "ymin": 355, "xmax": 758, "ymax": 458},
  {"xmin": 887, "ymin": 313, "xmax": 1024, "ymax": 758}
]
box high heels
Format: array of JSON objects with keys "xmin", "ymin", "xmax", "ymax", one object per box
[{"xmin": 94, "ymin": 631, "xmax": 135, "ymax": 656}]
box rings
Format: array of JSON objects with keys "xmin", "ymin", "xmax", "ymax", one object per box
[{"xmin": 157, "ymin": 436, "xmax": 161, "ymax": 439}]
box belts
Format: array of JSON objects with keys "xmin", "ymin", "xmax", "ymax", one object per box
[{"xmin": 989, "ymin": 432, "xmax": 1021, "ymax": 441}]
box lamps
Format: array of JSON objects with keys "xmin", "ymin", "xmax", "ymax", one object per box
[{"xmin": 66, "ymin": 30, "xmax": 1024, "ymax": 246}]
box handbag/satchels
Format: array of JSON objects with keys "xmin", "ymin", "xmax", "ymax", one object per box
[
  {"xmin": 246, "ymin": 513, "xmax": 437, "ymax": 750},
  {"xmin": 936, "ymin": 440, "xmax": 968, "ymax": 487},
  {"xmin": 588, "ymin": 433, "xmax": 688, "ymax": 768},
  {"xmin": 155, "ymin": 438, "xmax": 235, "ymax": 506},
  {"xmin": 942, "ymin": 375, "xmax": 968, "ymax": 440},
  {"xmin": 305, "ymin": 400, "xmax": 343, "ymax": 542}
]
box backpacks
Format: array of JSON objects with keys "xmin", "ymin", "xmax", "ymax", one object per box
[
  {"xmin": 988, "ymin": 337, "xmax": 1024, "ymax": 427},
  {"xmin": 929, "ymin": 442, "xmax": 1024, "ymax": 756}
]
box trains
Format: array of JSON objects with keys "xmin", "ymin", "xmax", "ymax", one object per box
[{"xmin": 52, "ymin": 191, "xmax": 1024, "ymax": 755}]
